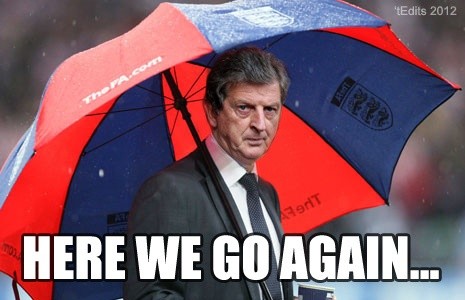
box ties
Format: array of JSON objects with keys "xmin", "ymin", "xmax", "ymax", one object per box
[{"xmin": 237, "ymin": 173, "xmax": 282, "ymax": 299}]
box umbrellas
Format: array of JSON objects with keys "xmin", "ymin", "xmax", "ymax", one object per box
[{"xmin": 0, "ymin": 1, "xmax": 462, "ymax": 299}]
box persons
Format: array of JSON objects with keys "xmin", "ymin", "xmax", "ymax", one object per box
[{"xmin": 122, "ymin": 48, "xmax": 336, "ymax": 300}]
